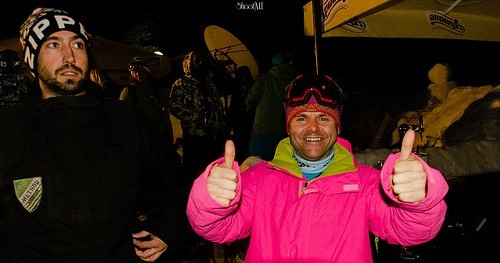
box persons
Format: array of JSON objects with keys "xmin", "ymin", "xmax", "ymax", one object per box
[
  {"xmin": 168, "ymin": 51, "xmax": 227, "ymax": 183},
  {"xmin": 0, "ymin": 4, "xmax": 193, "ymax": 263},
  {"xmin": 117, "ymin": 54, "xmax": 169, "ymax": 113},
  {"xmin": 0, "ymin": 48, "xmax": 32, "ymax": 117},
  {"xmin": 186, "ymin": 73, "xmax": 449, "ymax": 263},
  {"xmin": 88, "ymin": 66, "xmax": 105, "ymax": 87},
  {"xmin": 245, "ymin": 54, "xmax": 290, "ymax": 161},
  {"xmin": 224, "ymin": 64, "xmax": 256, "ymax": 148}
]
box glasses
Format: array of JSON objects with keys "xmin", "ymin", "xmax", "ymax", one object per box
[
  {"xmin": 128, "ymin": 61, "xmax": 144, "ymax": 71},
  {"xmin": 284, "ymin": 74, "xmax": 343, "ymax": 107}
]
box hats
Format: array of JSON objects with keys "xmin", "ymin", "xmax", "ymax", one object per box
[
  {"xmin": 0, "ymin": 49, "xmax": 20, "ymax": 73},
  {"xmin": 128, "ymin": 57, "xmax": 150, "ymax": 71},
  {"xmin": 272, "ymin": 54, "xmax": 287, "ymax": 65},
  {"xmin": 19, "ymin": 7, "xmax": 90, "ymax": 81},
  {"xmin": 284, "ymin": 74, "xmax": 343, "ymax": 134}
]
